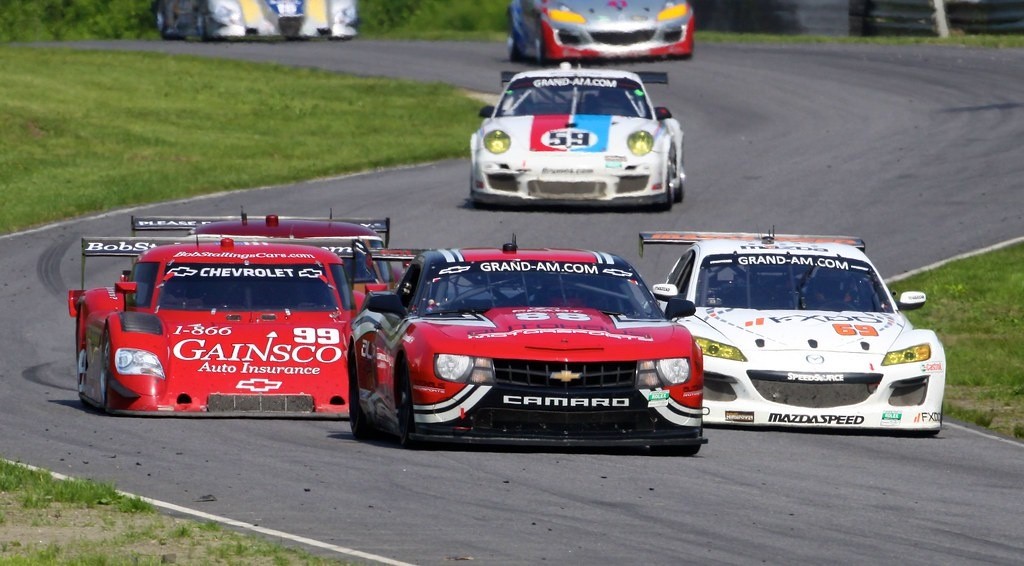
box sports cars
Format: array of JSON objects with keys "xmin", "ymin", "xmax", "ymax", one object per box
[
  {"xmin": 464, "ymin": 62, "xmax": 688, "ymax": 213},
  {"xmin": 638, "ymin": 228, "xmax": 949, "ymax": 437},
  {"xmin": 131, "ymin": 210, "xmax": 407, "ymax": 300},
  {"xmin": 346, "ymin": 242, "xmax": 710, "ymax": 457},
  {"xmin": 154, "ymin": 1, "xmax": 359, "ymax": 44},
  {"xmin": 504, "ymin": 0, "xmax": 695, "ymax": 67},
  {"xmin": 67, "ymin": 233, "xmax": 388, "ymax": 422}
]
